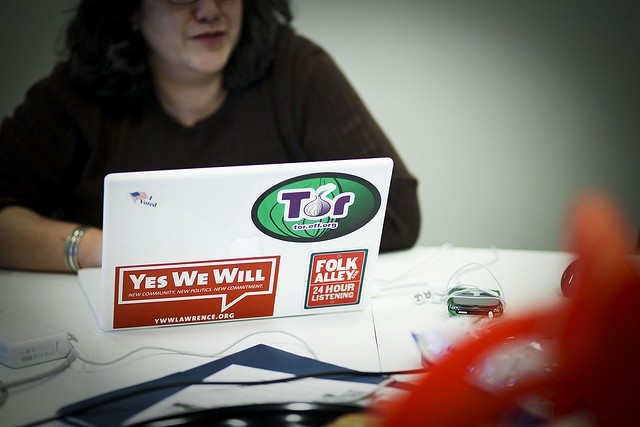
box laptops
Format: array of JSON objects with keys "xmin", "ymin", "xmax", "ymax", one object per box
[{"xmin": 76, "ymin": 156, "xmax": 394, "ymax": 332}]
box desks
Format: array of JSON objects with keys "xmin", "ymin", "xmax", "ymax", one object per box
[{"xmin": 1, "ymin": 246, "xmax": 639, "ymax": 426}]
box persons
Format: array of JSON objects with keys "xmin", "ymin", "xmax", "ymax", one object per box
[{"xmin": 1, "ymin": 0, "xmax": 419, "ymax": 274}]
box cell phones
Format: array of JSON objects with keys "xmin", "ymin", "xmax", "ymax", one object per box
[{"xmin": 449, "ymin": 289, "xmax": 504, "ymax": 319}]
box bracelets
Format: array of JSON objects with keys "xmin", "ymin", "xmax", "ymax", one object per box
[{"xmin": 63, "ymin": 223, "xmax": 92, "ymax": 274}]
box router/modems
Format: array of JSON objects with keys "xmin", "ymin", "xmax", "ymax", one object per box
[{"xmin": 0, "ymin": 320, "xmax": 75, "ymax": 369}]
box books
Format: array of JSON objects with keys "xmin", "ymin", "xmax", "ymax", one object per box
[{"xmin": 56, "ymin": 344, "xmax": 394, "ymax": 427}]
